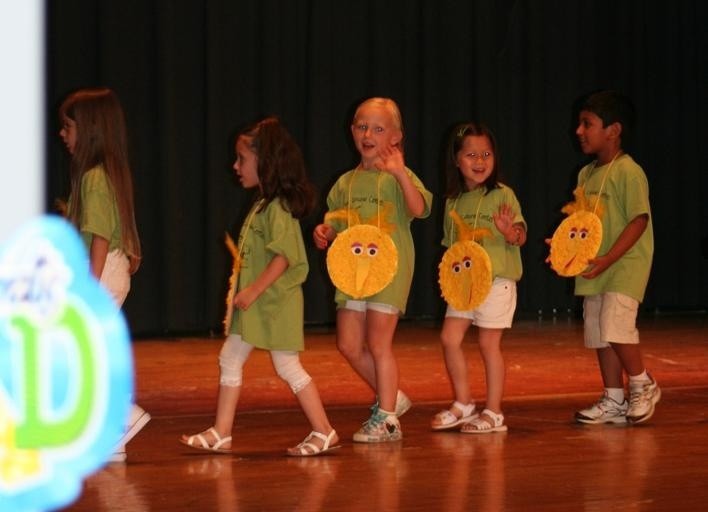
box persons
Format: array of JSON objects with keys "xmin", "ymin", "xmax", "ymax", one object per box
[
  {"xmin": 543, "ymin": 99, "xmax": 664, "ymax": 426},
  {"xmin": 176, "ymin": 115, "xmax": 344, "ymax": 453},
  {"xmin": 312, "ymin": 97, "xmax": 435, "ymax": 444},
  {"xmin": 429, "ymin": 124, "xmax": 529, "ymax": 436},
  {"xmin": 52, "ymin": 85, "xmax": 152, "ymax": 461}
]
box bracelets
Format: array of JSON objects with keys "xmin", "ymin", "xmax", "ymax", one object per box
[{"xmin": 506, "ymin": 229, "xmax": 521, "ymax": 247}]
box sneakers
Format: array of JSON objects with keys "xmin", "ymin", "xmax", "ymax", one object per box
[
  {"xmin": 575, "ymin": 397, "xmax": 628, "ymax": 425},
  {"xmin": 122, "ymin": 406, "xmax": 149, "ymax": 443},
  {"xmin": 626, "ymin": 372, "xmax": 661, "ymax": 425},
  {"xmin": 352, "ymin": 414, "xmax": 403, "ymax": 443},
  {"xmin": 370, "ymin": 390, "xmax": 411, "ymax": 419},
  {"xmin": 111, "ymin": 445, "xmax": 128, "ymax": 464}
]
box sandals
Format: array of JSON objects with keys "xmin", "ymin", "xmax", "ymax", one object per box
[
  {"xmin": 285, "ymin": 430, "xmax": 341, "ymax": 456},
  {"xmin": 178, "ymin": 428, "xmax": 233, "ymax": 454},
  {"xmin": 460, "ymin": 409, "xmax": 508, "ymax": 433},
  {"xmin": 431, "ymin": 401, "xmax": 480, "ymax": 429}
]
